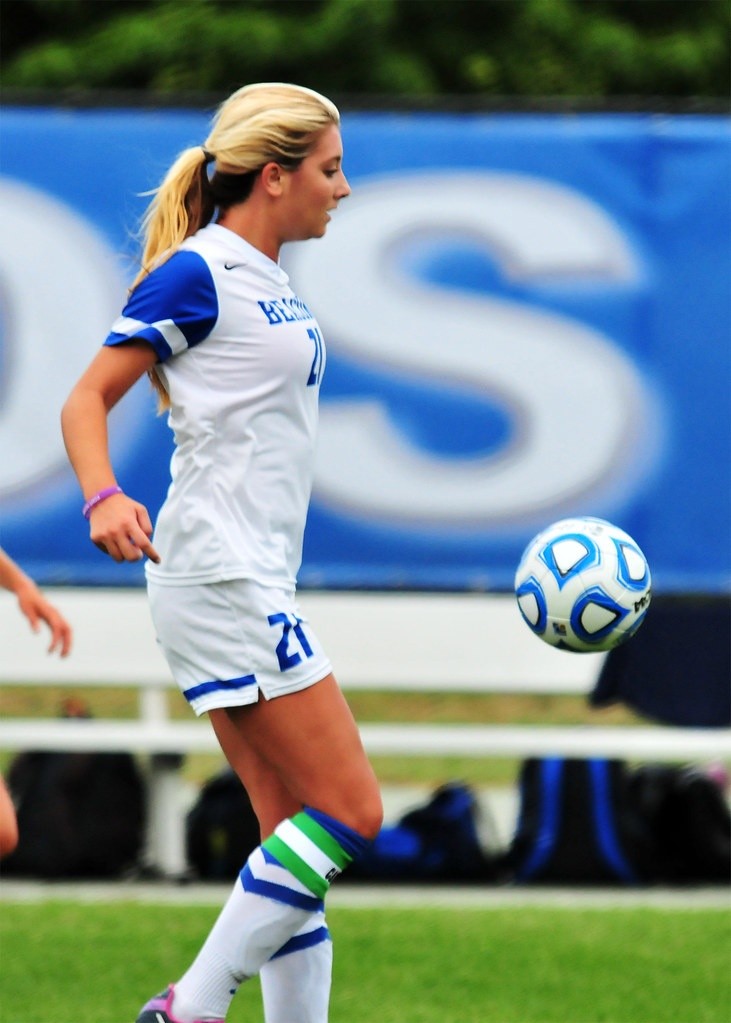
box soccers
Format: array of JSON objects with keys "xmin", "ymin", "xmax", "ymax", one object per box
[{"xmin": 513, "ymin": 515, "xmax": 654, "ymax": 654}]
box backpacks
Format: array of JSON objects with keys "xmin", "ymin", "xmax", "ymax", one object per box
[
  {"xmin": 362, "ymin": 782, "xmax": 489, "ymax": 883},
  {"xmin": 502, "ymin": 755, "xmax": 632, "ymax": 886}
]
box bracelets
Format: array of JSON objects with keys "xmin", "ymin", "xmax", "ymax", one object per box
[{"xmin": 84, "ymin": 487, "xmax": 122, "ymax": 519}]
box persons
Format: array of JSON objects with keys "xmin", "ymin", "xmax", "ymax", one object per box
[
  {"xmin": 61, "ymin": 83, "xmax": 385, "ymax": 1023},
  {"xmin": 0, "ymin": 549, "xmax": 73, "ymax": 854},
  {"xmin": 11, "ymin": 700, "xmax": 197, "ymax": 883}
]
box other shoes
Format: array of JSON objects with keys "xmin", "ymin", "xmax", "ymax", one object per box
[{"xmin": 134, "ymin": 984, "xmax": 224, "ymax": 1023}]
